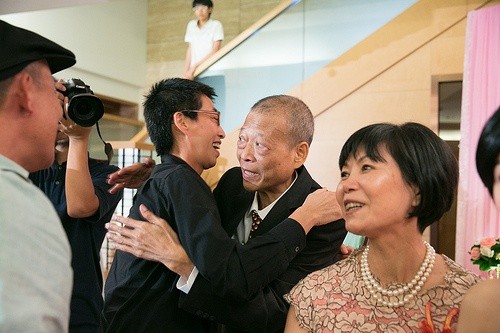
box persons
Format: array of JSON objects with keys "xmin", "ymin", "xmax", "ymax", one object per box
[
  {"xmin": 456, "ymin": 105, "xmax": 500, "ymax": 333},
  {"xmin": 183, "ymin": 0, "xmax": 223, "ymax": 80},
  {"xmin": 100, "ymin": 78, "xmax": 343, "ymax": 333},
  {"xmin": 104, "ymin": 95, "xmax": 348, "ymax": 333},
  {"xmin": 0, "ymin": 21, "xmax": 122, "ymax": 333},
  {"xmin": 283, "ymin": 122, "xmax": 482, "ymax": 333}
]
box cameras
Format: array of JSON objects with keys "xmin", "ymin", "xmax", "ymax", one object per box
[{"xmin": 56, "ymin": 78, "xmax": 105, "ymax": 128}]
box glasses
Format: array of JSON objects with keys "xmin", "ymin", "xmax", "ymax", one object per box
[
  {"xmin": 193, "ymin": 5, "xmax": 207, "ymax": 10},
  {"xmin": 180, "ymin": 110, "xmax": 220, "ymax": 126}
]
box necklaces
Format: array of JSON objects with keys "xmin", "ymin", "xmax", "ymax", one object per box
[{"xmin": 361, "ymin": 241, "xmax": 436, "ymax": 307}]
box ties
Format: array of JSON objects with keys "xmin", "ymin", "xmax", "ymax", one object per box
[{"xmin": 247, "ymin": 210, "xmax": 261, "ymax": 243}]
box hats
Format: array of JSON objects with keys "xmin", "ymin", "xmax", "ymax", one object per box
[{"xmin": 0, "ymin": 20, "xmax": 77, "ymax": 82}]
